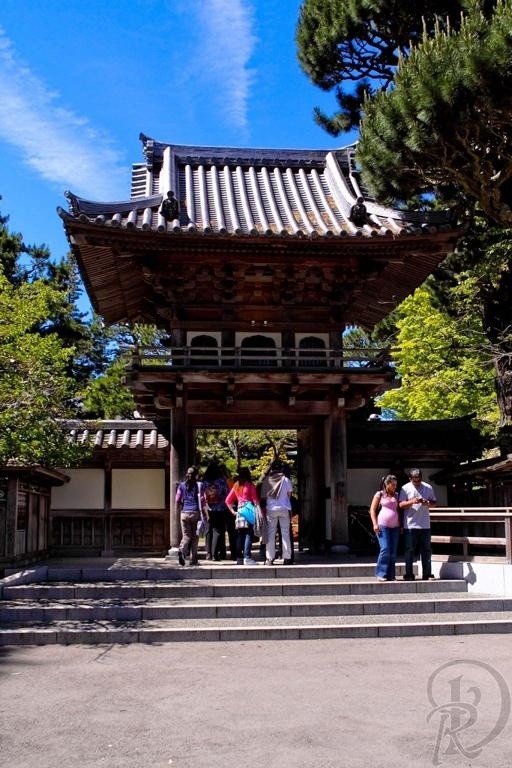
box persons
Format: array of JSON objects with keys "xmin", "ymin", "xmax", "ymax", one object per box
[
  {"xmin": 367, "ymin": 472, "xmax": 404, "ymax": 582},
  {"xmin": 174, "ymin": 457, "xmax": 296, "ymax": 567},
  {"xmin": 396, "ymin": 467, "xmax": 437, "ymax": 582}
]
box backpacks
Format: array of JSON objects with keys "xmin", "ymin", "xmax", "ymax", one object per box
[{"xmin": 204, "ymin": 484, "xmax": 220, "ymax": 506}]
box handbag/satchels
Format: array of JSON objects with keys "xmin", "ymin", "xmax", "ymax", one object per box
[
  {"xmin": 195, "ymin": 520, "xmax": 210, "ymax": 538},
  {"xmin": 368, "ymin": 502, "xmax": 381, "ymax": 517},
  {"xmin": 239, "ymin": 501, "xmax": 256, "ymax": 525}
]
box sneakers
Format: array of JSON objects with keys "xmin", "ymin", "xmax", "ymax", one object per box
[
  {"xmin": 188, "ymin": 561, "xmax": 199, "ymax": 566},
  {"xmin": 205, "ymin": 554, "xmax": 255, "ymax": 565},
  {"xmin": 265, "ymin": 558, "xmax": 291, "ymax": 565},
  {"xmin": 376, "ymin": 575, "xmax": 435, "ymax": 581},
  {"xmin": 177, "ymin": 550, "xmax": 185, "ymax": 566}
]
